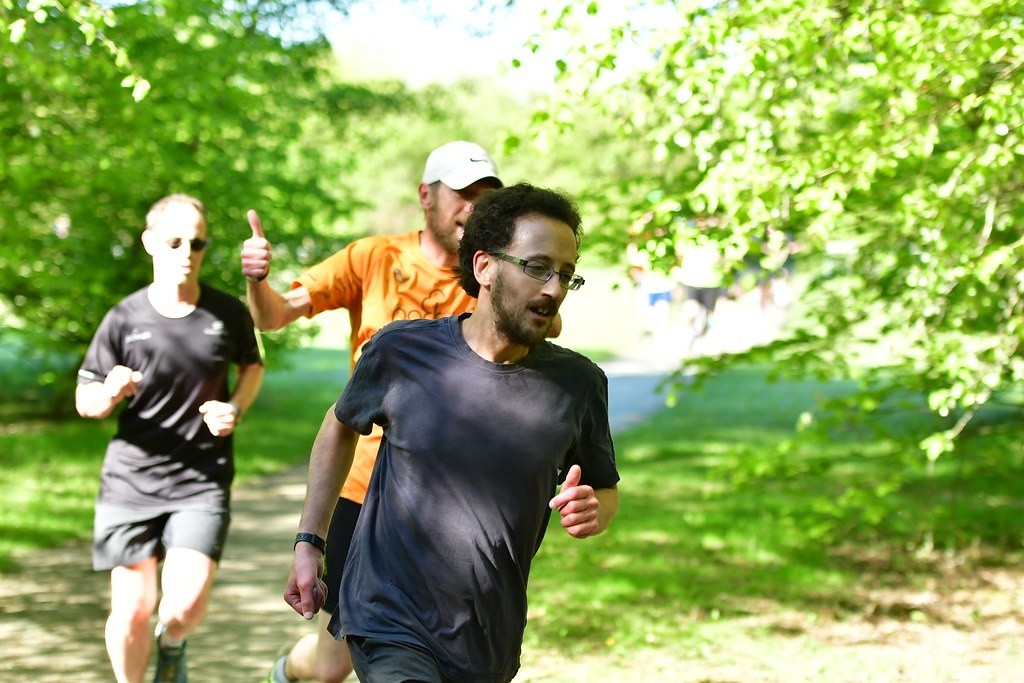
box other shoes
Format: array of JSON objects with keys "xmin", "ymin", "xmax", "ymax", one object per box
[{"xmin": 153, "ymin": 637, "xmax": 189, "ymax": 683}]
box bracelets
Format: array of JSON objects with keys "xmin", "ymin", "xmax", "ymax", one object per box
[
  {"xmin": 245, "ymin": 265, "xmax": 270, "ymax": 283},
  {"xmin": 293, "ymin": 532, "xmax": 326, "ymax": 556}
]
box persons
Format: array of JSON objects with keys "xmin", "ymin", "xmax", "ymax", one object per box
[
  {"xmin": 282, "ymin": 181, "xmax": 622, "ymax": 683},
  {"xmin": 240, "ymin": 139, "xmax": 562, "ymax": 683},
  {"xmin": 76, "ymin": 193, "xmax": 265, "ymax": 683}
]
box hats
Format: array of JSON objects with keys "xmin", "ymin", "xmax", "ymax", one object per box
[{"xmin": 423, "ymin": 141, "xmax": 508, "ymax": 190}]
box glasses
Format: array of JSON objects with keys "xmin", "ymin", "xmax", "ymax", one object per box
[
  {"xmin": 165, "ymin": 235, "xmax": 208, "ymax": 253},
  {"xmin": 492, "ymin": 250, "xmax": 585, "ymax": 291}
]
